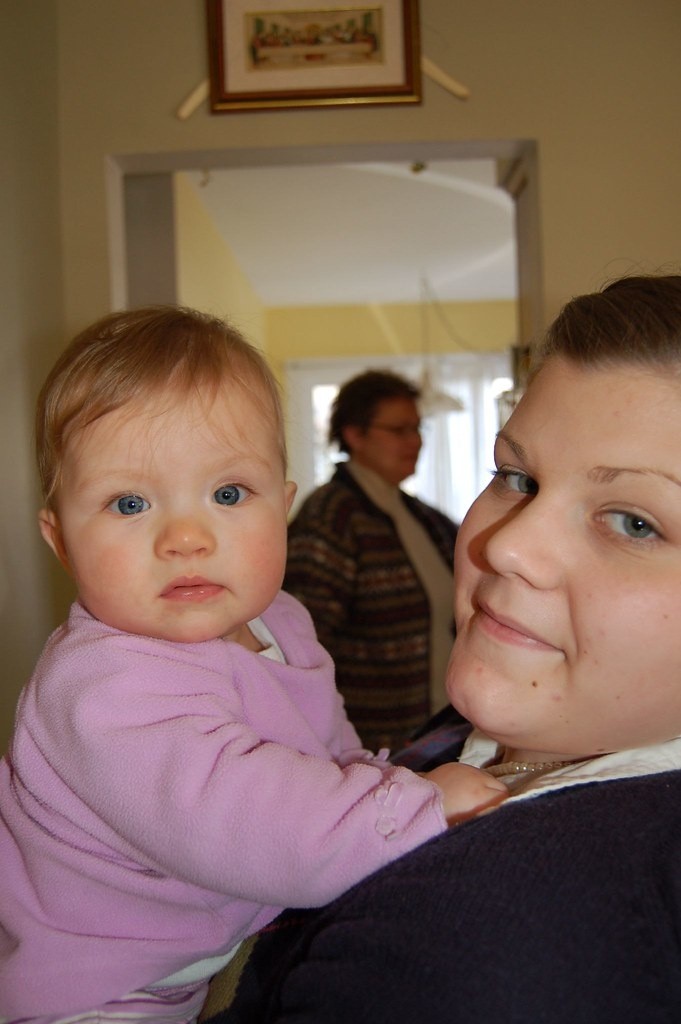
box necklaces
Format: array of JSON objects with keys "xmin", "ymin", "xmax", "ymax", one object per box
[{"xmin": 480, "ymin": 761, "xmax": 585, "ymax": 778}]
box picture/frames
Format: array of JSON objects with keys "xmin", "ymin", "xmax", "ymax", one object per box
[{"xmin": 206, "ymin": 0, "xmax": 424, "ymax": 113}]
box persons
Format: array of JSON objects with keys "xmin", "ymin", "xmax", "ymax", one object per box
[
  {"xmin": 194, "ymin": 272, "xmax": 680, "ymax": 1024},
  {"xmin": 0, "ymin": 303, "xmax": 508, "ymax": 1024},
  {"xmin": 282, "ymin": 369, "xmax": 473, "ymax": 774}
]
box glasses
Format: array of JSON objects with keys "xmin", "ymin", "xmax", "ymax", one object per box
[{"xmin": 369, "ymin": 420, "xmax": 420, "ymax": 442}]
